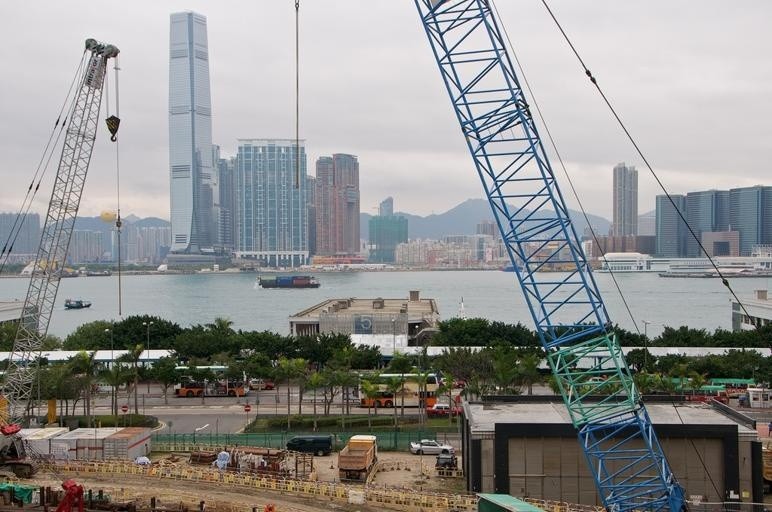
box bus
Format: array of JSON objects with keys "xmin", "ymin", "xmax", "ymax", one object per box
[
  {"xmin": 173, "ymin": 365, "xmax": 249, "ymax": 397},
  {"xmin": 639, "ymin": 376, "xmax": 693, "ymax": 394},
  {"xmin": 672, "ymin": 385, "xmax": 730, "ymax": 404},
  {"xmin": 707, "ymin": 378, "xmax": 757, "ymax": 398},
  {"xmin": 359, "ymin": 373, "xmax": 439, "ymax": 408}
]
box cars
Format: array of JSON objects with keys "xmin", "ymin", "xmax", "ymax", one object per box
[
  {"xmin": 579, "ymin": 376, "xmax": 611, "ymax": 391},
  {"xmin": 439, "ymin": 378, "xmax": 465, "ymax": 389},
  {"xmin": 410, "ymin": 439, "xmax": 456, "ymax": 455},
  {"xmin": 435, "ymin": 453, "xmax": 458, "ymax": 475},
  {"xmin": 425, "ymin": 403, "xmax": 461, "ymax": 418},
  {"xmin": 249, "ymin": 378, "xmax": 275, "ymax": 390}
]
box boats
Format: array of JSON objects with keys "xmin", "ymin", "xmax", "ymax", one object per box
[
  {"xmin": 64, "ymin": 297, "xmax": 92, "ymax": 310},
  {"xmin": 258, "ymin": 275, "xmax": 321, "ymax": 289},
  {"xmin": 86, "ymin": 270, "xmax": 113, "ymax": 277}
]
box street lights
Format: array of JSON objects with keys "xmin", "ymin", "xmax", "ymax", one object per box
[
  {"xmin": 104, "ymin": 328, "xmax": 114, "ymax": 416},
  {"xmin": 391, "ymin": 319, "xmax": 396, "ymax": 360},
  {"xmin": 142, "ymin": 321, "xmax": 155, "ymax": 394},
  {"xmin": 415, "ymin": 325, "xmax": 419, "ymax": 374},
  {"xmin": 641, "ymin": 319, "xmax": 651, "ymax": 372}
]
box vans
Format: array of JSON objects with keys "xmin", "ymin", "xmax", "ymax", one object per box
[{"xmin": 285, "ymin": 435, "xmax": 332, "ymax": 457}]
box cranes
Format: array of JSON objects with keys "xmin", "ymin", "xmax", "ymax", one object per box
[{"xmin": 0, "ymin": 38, "xmax": 122, "ymax": 479}]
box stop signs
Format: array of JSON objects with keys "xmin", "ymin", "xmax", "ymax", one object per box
[
  {"xmin": 122, "ymin": 405, "xmax": 128, "ymax": 412},
  {"xmin": 244, "ymin": 404, "xmax": 251, "ymax": 412}
]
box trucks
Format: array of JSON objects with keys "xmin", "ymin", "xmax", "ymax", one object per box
[
  {"xmin": 339, "ymin": 434, "xmax": 377, "ymax": 483},
  {"xmin": 189, "ymin": 446, "xmax": 288, "ymax": 479}
]
